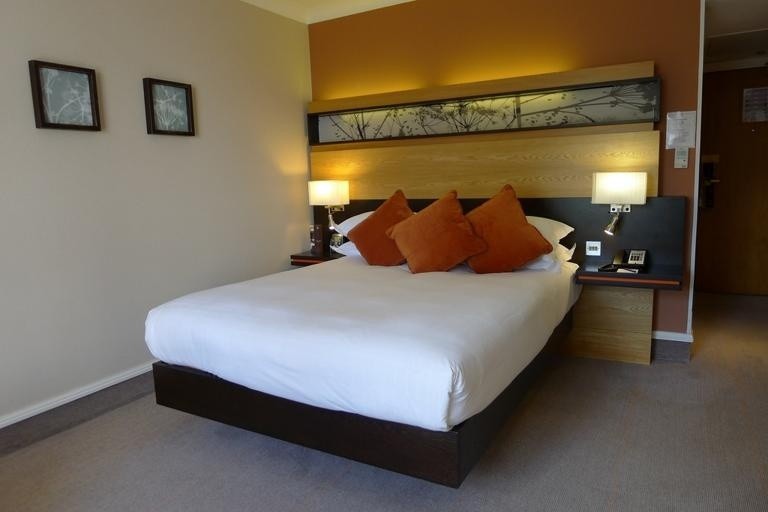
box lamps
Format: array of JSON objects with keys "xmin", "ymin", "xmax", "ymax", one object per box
[
  {"xmin": 591, "ymin": 172, "xmax": 648, "ymax": 209},
  {"xmin": 328, "ymin": 209, "xmax": 338, "ymax": 230},
  {"xmin": 308, "ymin": 180, "xmax": 351, "ymax": 208},
  {"xmin": 604, "ymin": 210, "xmax": 621, "ymax": 236}
]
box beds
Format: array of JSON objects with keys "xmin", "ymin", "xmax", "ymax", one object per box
[{"xmin": 143, "ymin": 196, "xmax": 688, "ymax": 490}]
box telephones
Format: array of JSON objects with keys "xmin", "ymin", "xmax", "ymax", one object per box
[{"xmin": 612, "ymin": 249, "xmax": 647, "ymax": 268}]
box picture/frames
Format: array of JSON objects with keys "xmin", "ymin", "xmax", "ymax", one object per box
[
  {"xmin": 28, "ymin": 60, "xmax": 100, "ymax": 132},
  {"xmin": 143, "ymin": 77, "xmax": 195, "ymax": 137}
]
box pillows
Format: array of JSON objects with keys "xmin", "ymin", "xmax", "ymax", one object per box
[{"xmin": 331, "ymin": 184, "xmax": 578, "ymax": 276}]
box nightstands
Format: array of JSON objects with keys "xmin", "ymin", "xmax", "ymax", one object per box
[
  {"xmin": 289, "ymin": 247, "xmax": 344, "ymax": 267},
  {"xmin": 568, "ymin": 265, "xmax": 683, "ymax": 368}
]
